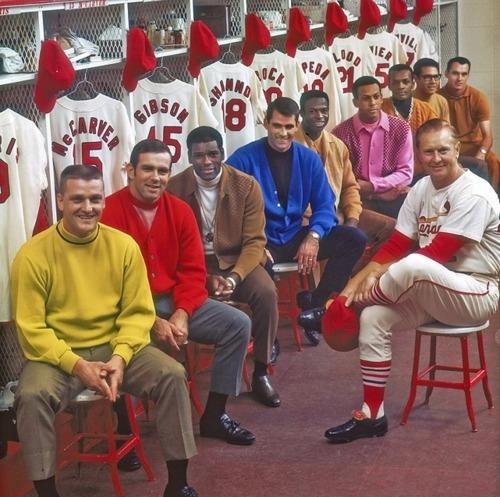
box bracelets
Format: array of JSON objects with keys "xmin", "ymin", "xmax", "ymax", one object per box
[{"xmin": 227, "ymin": 275, "xmax": 237, "ymax": 291}]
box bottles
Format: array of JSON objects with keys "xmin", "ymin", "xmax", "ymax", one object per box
[{"xmin": 130, "ymin": 11, "xmax": 187, "ymax": 50}]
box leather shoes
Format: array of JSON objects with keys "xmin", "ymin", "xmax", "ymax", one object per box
[
  {"xmin": 251, "ymin": 370, "xmax": 283, "ymax": 407},
  {"xmin": 202, "ymin": 407, "xmax": 264, "ymax": 445},
  {"xmin": 323, "ymin": 410, "xmax": 390, "ymax": 445},
  {"xmin": 113, "ymin": 425, "xmax": 148, "ymax": 473},
  {"xmin": 294, "ymin": 286, "xmax": 329, "ymax": 345},
  {"xmin": 269, "ymin": 337, "xmax": 281, "ymax": 366}
]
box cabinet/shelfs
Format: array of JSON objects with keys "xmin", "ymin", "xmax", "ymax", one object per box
[{"xmin": 2, "ymin": 0, "xmax": 458, "ymax": 408}]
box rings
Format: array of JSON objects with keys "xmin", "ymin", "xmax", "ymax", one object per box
[{"xmin": 309, "ymin": 258, "xmax": 313, "ymax": 260}]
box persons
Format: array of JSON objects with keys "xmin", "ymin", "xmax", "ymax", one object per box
[
  {"xmin": 97, "ymin": 137, "xmax": 260, "ymax": 472},
  {"xmin": 165, "ymin": 124, "xmax": 284, "ymax": 412},
  {"xmin": 433, "ymin": 55, "xmax": 500, "ymax": 190},
  {"xmin": 6, "ymin": 162, "xmax": 201, "ymax": 496},
  {"xmin": 225, "ymin": 96, "xmax": 367, "ymax": 367},
  {"xmin": 296, "ymin": 90, "xmax": 399, "ymax": 277},
  {"xmin": 410, "ymin": 55, "xmax": 488, "ymax": 181},
  {"xmin": 295, "ymin": 114, "xmax": 500, "ymax": 445},
  {"xmin": 382, "ymin": 63, "xmax": 437, "ymax": 183},
  {"xmin": 331, "ymin": 75, "xmax": 418, "ymax": 218}
]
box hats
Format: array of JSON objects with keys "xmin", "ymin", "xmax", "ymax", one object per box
[{"xmin": 323, "ymin": 293, "xmax": 363, "ymax": 353}]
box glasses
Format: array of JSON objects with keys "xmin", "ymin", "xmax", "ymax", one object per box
[{"xmin": 416, "ymin": 73, "xmax": 443, "ymax": 81}]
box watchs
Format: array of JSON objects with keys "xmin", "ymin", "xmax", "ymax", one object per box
[
  {"xmin": 479, "ymin": 147, "xmax": 489, "ymax": 154},
  {"xmin": 307, "ymin": 230, "xmax": 321, "ymax": 241}
]
